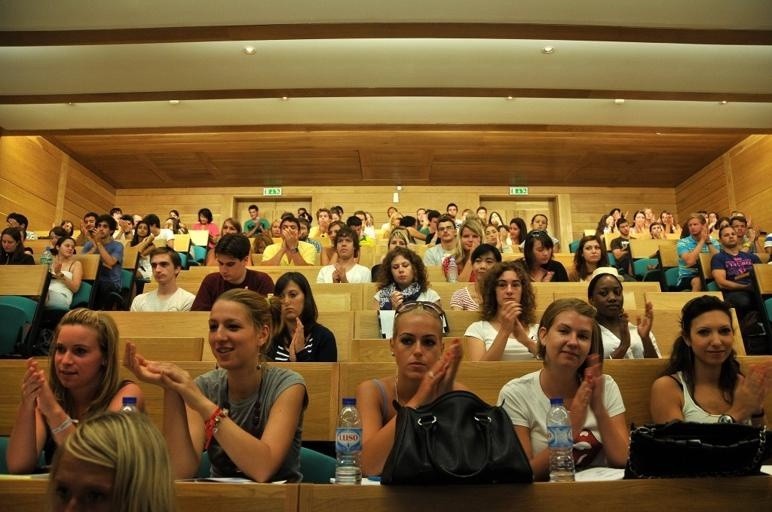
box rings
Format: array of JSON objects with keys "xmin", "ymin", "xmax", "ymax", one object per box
[{"xmin": 428, "ymin": 371, "xmax": 434, "ymax": 378}]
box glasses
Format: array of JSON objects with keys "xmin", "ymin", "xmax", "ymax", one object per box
[
  {"xmin": 395, "ymin": 301, "xmax": 444, "ymax": 319},
  {"xmin": 528, "ymin": 230, "xmax": 548, "ymax": 238}
]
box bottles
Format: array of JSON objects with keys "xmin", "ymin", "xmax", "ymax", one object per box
[
  {"xmin": 40, "ymin": 246, "xmax": 53, "ymax": 265},
  {"xmin": 118, "ymin": 395, "xmax": 142, "ymax": 419},
  {"xmin": 332, "ymin": 395, "xmax": 364, "ymax": 488},
  {"xmin": 445, "ymin": 256, "xmax": 459, "ymax": 282},
  {"xmin": 545, "ymin": 398, "xmax": 577, "ymax": 483}
]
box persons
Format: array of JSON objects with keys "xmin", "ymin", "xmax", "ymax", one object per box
[
  {"xmin": 354, "ymin": 301, "xmax": 470, "ymax": 476},
  {"xmin": 650, "ymin": 295, "xmax": 772, "ymax": 427},
  {"xmin": 497, "ymin": 298, "xmax": 629, "ymax": 478},
  {"xmin": 5, "ymin": 307, "xmax": 145, "ymax": 474},
  {"xmin": 122, "ymin": 289, "xmax": 308, "ymax": 483},
  {"xmin": 46, "ymin": 411, "xmax": 177, "ymax": 512}
]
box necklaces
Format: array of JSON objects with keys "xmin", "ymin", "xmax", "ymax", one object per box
[
  {"xmin": 224, "ymin": 378, "xmax": 262, "ymax": 434},
  {"xmin": 394, "ymin": 377, "xmax": 399, "ymax": 406}
]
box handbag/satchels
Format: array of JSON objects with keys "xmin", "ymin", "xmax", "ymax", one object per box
[
  {"xmin": 14, "ymin": 322, "xmax": 53, "ymax": 356},
  {"xmin": 382, "ymin": 390, "xmax": 534, "ymax": 486},
  {"xmin": 625, "ymin": 421, "xmax": 766, "ymax": 478}
]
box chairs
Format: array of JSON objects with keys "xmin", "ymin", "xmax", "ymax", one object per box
[{"xmin": 0, "ymin": 226, "xmax": 770, "ymax": 512}]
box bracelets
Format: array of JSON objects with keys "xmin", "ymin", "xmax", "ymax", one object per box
[
  {"xmin": 718, "ymin": 414, "xmax": 735, "ymax": 424},
  {"xmin": 750, "ymin": 408, "xmax": 764, "ymax": 418},
  {"xmin": 203, "ymin": 406, "xmax": 229, "ymax": 452},
  {"xmin": 52, "ymin": 415, "xmax": 79, "ymax": 435}
]
